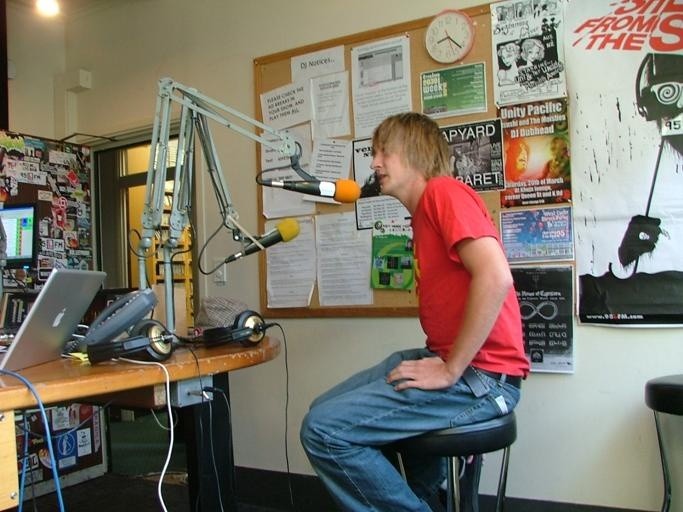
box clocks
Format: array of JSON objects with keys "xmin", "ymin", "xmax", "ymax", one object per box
[{"xmin": 424, "ymin": 9, "xmax": 475, "ymax": 65}]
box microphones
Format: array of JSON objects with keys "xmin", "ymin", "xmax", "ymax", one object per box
[
  {"xmin": 262, "ymin": 178, "xmax": 362, "ymax": 203},
  {"xmin": 225, "ymin": 218, "xmax": 300, "ymax": 265}
]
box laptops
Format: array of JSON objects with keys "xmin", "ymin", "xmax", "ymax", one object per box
[{"xmin": 0, "ymin": 269, "xmax": 107, "ymax": 376}]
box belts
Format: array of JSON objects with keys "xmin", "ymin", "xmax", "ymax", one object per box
[{"xmin": 460, "ymin": 364, "xmax": 523, "ymax": 399}]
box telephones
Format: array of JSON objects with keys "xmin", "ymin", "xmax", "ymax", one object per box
[{"xmin": 85, "ymin": 288, "xmax": 158, "ymax": 345}]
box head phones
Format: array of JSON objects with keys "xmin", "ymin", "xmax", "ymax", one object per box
[
  {"xmin": 203, "ymin": 310, "xmax": 274, "ymax": 348},
  {"xmin": 88, "ymin": 320, "xmax": 174, "ymax": 365},
  {"xmin": 633, "ymin": 53, "xmax": 661, "ymax": 123}
]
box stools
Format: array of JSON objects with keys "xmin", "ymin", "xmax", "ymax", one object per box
[{"xmin": 388, "ymin": 409, "xmax": 515, "ymax": 512}]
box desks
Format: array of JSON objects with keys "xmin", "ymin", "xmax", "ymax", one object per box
[{"xmin": 1, "ymin": 324, "xmax": 281, "ymax": 512}]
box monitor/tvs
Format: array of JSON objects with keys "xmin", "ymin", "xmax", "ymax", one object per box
[{"xmin": 0, "ymin": 203, "xmax": 38, "ymax": 265}]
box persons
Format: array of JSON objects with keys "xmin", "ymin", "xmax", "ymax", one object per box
[
  {"xmin": 517, "ymin": 39, "xmax": 547, "ymax": 73},
  {"xmin": 492, "ymin": 41, "xmax": 521, "ymax": 86},
  {"xmin": 296, "ymin": 111, "xmax": 527, "ymax": 510}
]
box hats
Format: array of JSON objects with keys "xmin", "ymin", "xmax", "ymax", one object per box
[{"xmin": 193, "ymin": 296, "xmax": 248, "ymax": 338}]
